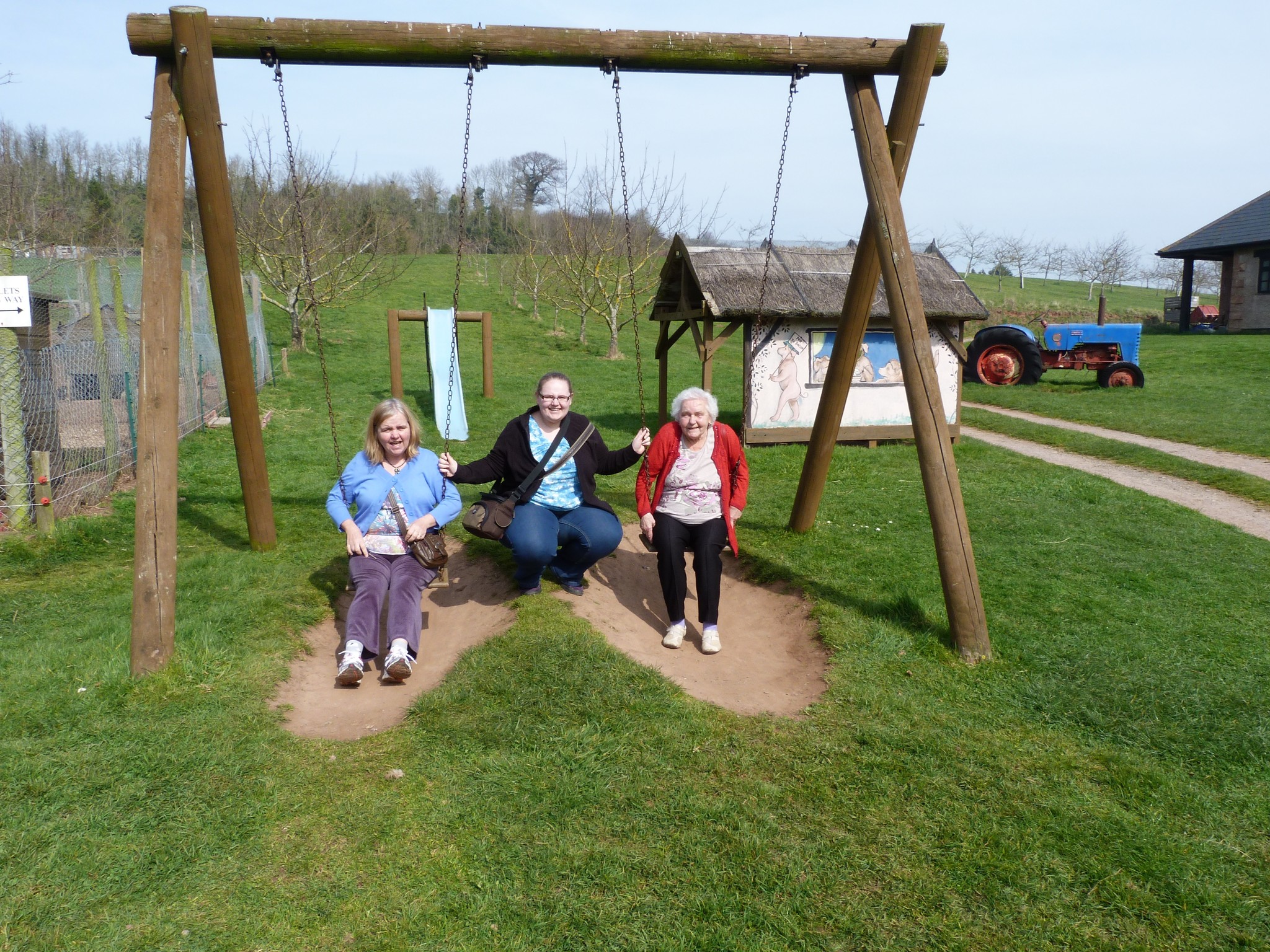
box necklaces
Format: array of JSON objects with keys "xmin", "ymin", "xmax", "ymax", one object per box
[{"xmin": 383, "ymin": 453, "xmax": 407, "ymax": 475}]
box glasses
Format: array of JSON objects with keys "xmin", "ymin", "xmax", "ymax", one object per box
[{"xmin": 538, "ymin": 392, "xmax": 571, "ymax": 403}]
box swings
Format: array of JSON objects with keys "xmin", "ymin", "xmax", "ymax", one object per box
[
  {"xmin": 274, "ymin": 57, "xmax": 473, "ymax": 567},
  {"xmin": 609, "ymin": 70, "xmax": 799, "ymax": 557}
]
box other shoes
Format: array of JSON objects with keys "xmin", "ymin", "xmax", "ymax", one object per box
[
  {"xmin": 518, "ymin": 576, "xmax": 541, "ymax": 595},
  {"xmin": 550, "ymin": 567, "xmax": 584, "ymax": 596}
]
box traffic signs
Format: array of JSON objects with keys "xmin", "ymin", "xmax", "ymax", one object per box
[{"xmin": 0, "ymin": 275, "xmax": 33, "ymax": 328}]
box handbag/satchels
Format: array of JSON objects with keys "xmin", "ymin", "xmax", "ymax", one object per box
[
  {"xmin": 462, "ymin": 500, "xmax": 515, "ymax": 540},
  {"xmin": 408, "ymin": 533, "xmax": 448, "ymax": 569}
]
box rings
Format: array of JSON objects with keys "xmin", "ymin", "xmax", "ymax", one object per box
[{"xmin": 418, "ymin": 534, "xmax": 422, "ymax": 537}]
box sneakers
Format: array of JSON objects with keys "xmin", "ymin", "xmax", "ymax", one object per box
[
  {"xmin": 382, "ymin": 649, "xmax": 418, "ymax": 683},
  {"xmin": 701, "ymin": 629, "xmax": 722, "ymax": 654},
  {"xmin": 663, "ymin": 623, "xmax": 688, "ymax": 648},
  {"xmin": 337, "ymin": 651, "xmax": 364, "ymax": 686}
]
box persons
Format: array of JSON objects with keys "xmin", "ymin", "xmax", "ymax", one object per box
[
  {"xmin": 325, "ymin": 399, "xmax": 463, "ymax": 687},
  {"xmin": 437, "ymin": 371, "xmax": 650, "ymax": 597},
  {"xmin": 636, "ymin": 387, "xmax": 749, "ymax": 652}
]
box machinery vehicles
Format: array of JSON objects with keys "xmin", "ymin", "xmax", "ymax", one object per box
[{"xmin": 964, "ymin": 294, "xmax": 1145, "ymax": 390}]
